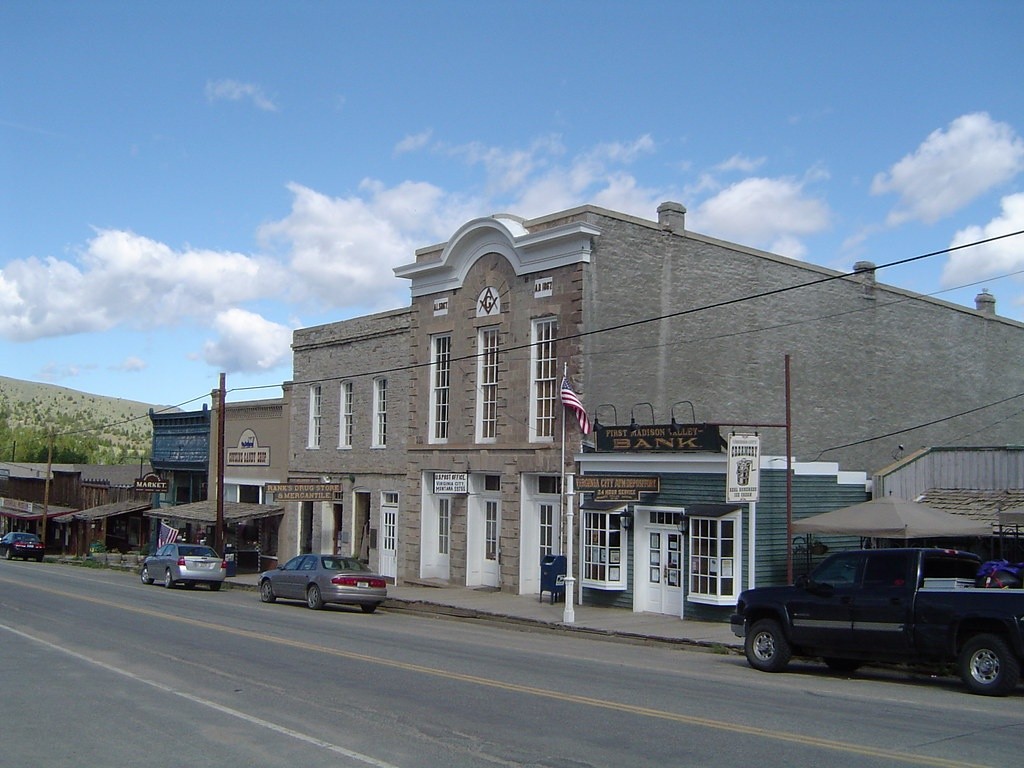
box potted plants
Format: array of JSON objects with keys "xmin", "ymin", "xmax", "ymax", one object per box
[{"xmin": 89, "ymin": 541, "xmax": 107, "ymax": 562}]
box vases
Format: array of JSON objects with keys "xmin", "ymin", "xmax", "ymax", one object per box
[
  {"xmin": 107, "ymin": 552, "xmax": 121, "ymax": 563},
  {"xmin": 122, "ymin": 555, "xmax": 138, "ymax": 565}
]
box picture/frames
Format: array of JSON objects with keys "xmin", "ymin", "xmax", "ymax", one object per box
[{"xmin": 610, "ymin": 550, "xmax": 619, "ymax": 563}]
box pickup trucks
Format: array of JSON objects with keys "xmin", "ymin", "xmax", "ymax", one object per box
[{"xmin": 730, "ymin": 546, "xmax": 1024, "ymax": 697}]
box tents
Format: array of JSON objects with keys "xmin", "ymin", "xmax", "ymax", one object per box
[{"xmin": 790, "ymin": 493, "xmax": 997, "ymax": 580}]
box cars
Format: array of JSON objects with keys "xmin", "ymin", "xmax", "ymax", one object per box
[
  {"xmin": 0, "ymin": 531, "xmax": 45, "ymax": 563},
  {"xmin": 257, "ymin": 554, "xmax": 388, "ymax": 614},
  {"xmin": 140, "ymin": 542, "xmax": 228, "ymax": 590}
]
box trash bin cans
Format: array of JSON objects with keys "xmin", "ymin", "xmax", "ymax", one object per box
[{"xmin": 539, "ymin": 554, "xmax": 566, "ymax": 604}]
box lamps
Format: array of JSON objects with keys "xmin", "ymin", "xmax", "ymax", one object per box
[
  {"xmin": 593, "ymin": 404, "xmax": 617, "ymax": 431},
  {"xmin": 892, "ymin": 444, "xmax": 904, "ymax": 462},
  {"xmin": 669, "ymin": 401, "xmax": 696, "ymax": 433},
  {"xmin": 620, "ymin": 509, "xmax": 631, "ymax": 531},
  {"xmin": 628, "ymin": 402, "xmax": 655, "ymax": 434},
  {"xmin": 677, "ymin": 512, "xmax": 688, "ymax": 536},
  {"xmin": 320, "ymin": 474, "xmax": 332, "ymax": 486}
]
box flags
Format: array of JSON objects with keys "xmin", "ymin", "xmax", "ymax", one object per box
[
  {"xmin": 158, "ymin": 523, "xmax": 178, "ymax": 547},
  {"xmin": 192, "ymin": 524, "xmax": 202, "ymax": 544},
  {"xmin": 559, "ymin": 376, "xmax": 591, "ymax": 435}
]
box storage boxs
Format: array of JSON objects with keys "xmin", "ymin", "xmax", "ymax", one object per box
[{"xmin": 923, "ymin": 578, "xmax": 975, "ymax": 589}]
